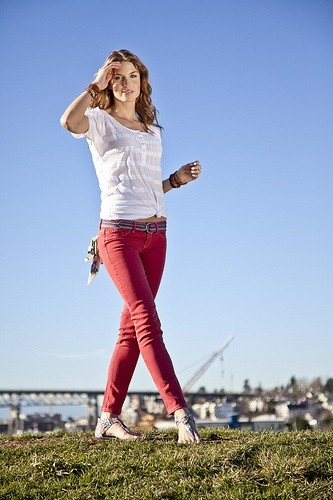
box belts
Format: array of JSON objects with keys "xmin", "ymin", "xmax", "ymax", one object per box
[{"xmin": 100, "ymin": 220, "xmax": 166, "ymax": 235}]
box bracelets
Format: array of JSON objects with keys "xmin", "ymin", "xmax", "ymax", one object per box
[
  {"xmin": 169, "ymin": 171, "xmax": 187, "ymax": 189},
  {"xmin": 86, "ymin": 84, "xmax": 101, "ymax": 99}
]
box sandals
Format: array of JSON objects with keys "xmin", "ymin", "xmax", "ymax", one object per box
[
  {"xmin": 95, "ymin": 418, "xmax": 142, "ymax": 441},
  {"xmin": 174, "ymin": 407, "xmax": 202, "ymax": 446}
]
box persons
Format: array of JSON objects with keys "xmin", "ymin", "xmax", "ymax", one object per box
[{"xmin": 60, "ymin": 50, "xmax": 204, "ymax": 445}]
